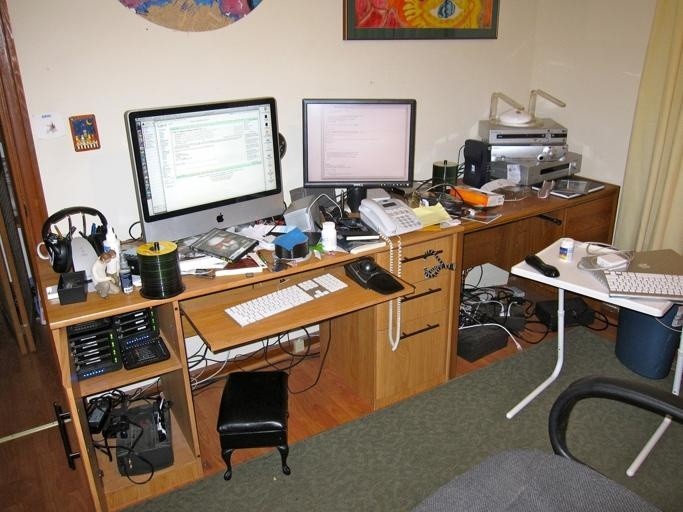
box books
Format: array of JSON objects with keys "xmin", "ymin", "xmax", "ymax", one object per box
[{"xmin": 177, "ymin": 227, "xmax": 268, "ymax": 278}]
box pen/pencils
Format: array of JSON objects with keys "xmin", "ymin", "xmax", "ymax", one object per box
[{"xmin": 256, "ymin": 250, "xmax": 268, "ymax": 264}]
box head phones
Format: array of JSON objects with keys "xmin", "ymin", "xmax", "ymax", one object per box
[{"xmin": 42, "ymin": 207, "xmax": 108, "ymax": 273}]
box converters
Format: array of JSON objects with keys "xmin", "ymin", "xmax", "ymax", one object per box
[
  {"xmin": 597, "ymin": 254, "xmax": 628, "ymax": 270},
  {"xmin": 88, "ymin": 401, "xmax": 110, "ymax": 433}
]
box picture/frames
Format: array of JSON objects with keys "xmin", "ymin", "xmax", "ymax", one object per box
[{"xmin": 342, "ymin": 0, "xmax": 500, "ymax": 40}]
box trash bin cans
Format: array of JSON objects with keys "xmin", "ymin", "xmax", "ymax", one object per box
[{"xmin": 615, "ymin": 304, "xmax": 683, "ymax": 379}]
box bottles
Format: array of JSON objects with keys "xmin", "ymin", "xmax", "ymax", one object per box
[
  {"xmin": 320, "ymin": 221, "xmax": 338, "ymax": 251},
  {"xmin": 119, "ymin": 252, "xmax": 134, "ymax": 295},
  {"xmin": 558, "ymin": 237, "xmax": 575, "ymax": 264},
  {"xmin": 102, "ymin": 222, "xmax": 122, "ymax": 275}
]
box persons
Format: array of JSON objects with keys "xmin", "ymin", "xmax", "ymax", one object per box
[{"xmin": 90, "ymin": 249, "xmax": 121, "ymax": 300}]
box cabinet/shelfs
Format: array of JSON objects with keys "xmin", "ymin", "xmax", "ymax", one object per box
[
  {"xmin": 54, "ymin": 304, "xmax": 203, "ymax": 512},
  {"xmin": 373, "ymin": 233, "xmax": 460, "ymax": 410},
  {"xmin": 464, "ymin": 182, "xmax": 620, "ymax": 326}
]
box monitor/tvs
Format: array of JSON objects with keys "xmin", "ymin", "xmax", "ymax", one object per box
[
  {"xmin": 124, "ymin": 97, "xmax": 285, "ymax": 244},
  {"xmin": 302, "ymin": 99, "xmax": 416, "ymax": 213}
]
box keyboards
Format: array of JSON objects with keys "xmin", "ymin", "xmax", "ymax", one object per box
[
  {"xmin": 604, "ymin": 269, "xmax": 683, "ymax": 304},
  {"xmin": 224, "ymin": 273, "xmax": 348, "ymax": 327}
]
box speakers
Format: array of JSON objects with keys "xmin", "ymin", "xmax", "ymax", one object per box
[{"xmin": 463, "ymin": 139, "xmax": 491, "ymax": 189}]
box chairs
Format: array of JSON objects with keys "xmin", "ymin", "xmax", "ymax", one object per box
[{"xmin": 406, "ymin": 373, "xmax": 683, "ymax": 512}]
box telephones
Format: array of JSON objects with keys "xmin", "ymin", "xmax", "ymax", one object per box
[{"xmin": 359, "ymin": 197, "xmax": 422, "ymax": 237}]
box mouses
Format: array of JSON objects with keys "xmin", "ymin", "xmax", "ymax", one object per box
[{"xmin": 361, "ymin": 261, "xmax": 375, "ymax": 274}]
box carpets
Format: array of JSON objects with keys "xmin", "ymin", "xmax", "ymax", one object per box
[{"xmin": 107, "ymin": 323, "xmax": 683, "ymax": 512}]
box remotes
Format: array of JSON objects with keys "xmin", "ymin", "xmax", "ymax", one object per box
[{"xmin": 525, "ymin": 254, "xmax": 560, "ymax": 277}]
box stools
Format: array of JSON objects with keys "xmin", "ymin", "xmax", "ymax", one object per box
[{"xmin": 218, "ymin": 370, "xmax": 291, "ymax": 481}]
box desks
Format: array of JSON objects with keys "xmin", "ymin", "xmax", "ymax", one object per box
[
  {"xmin": 506, "ymin": 236, "xmax": 683, "ymax": 478},
  {"xmin": 28, "ymin": 207, "xmax": 463, "ymax": 512}
]
